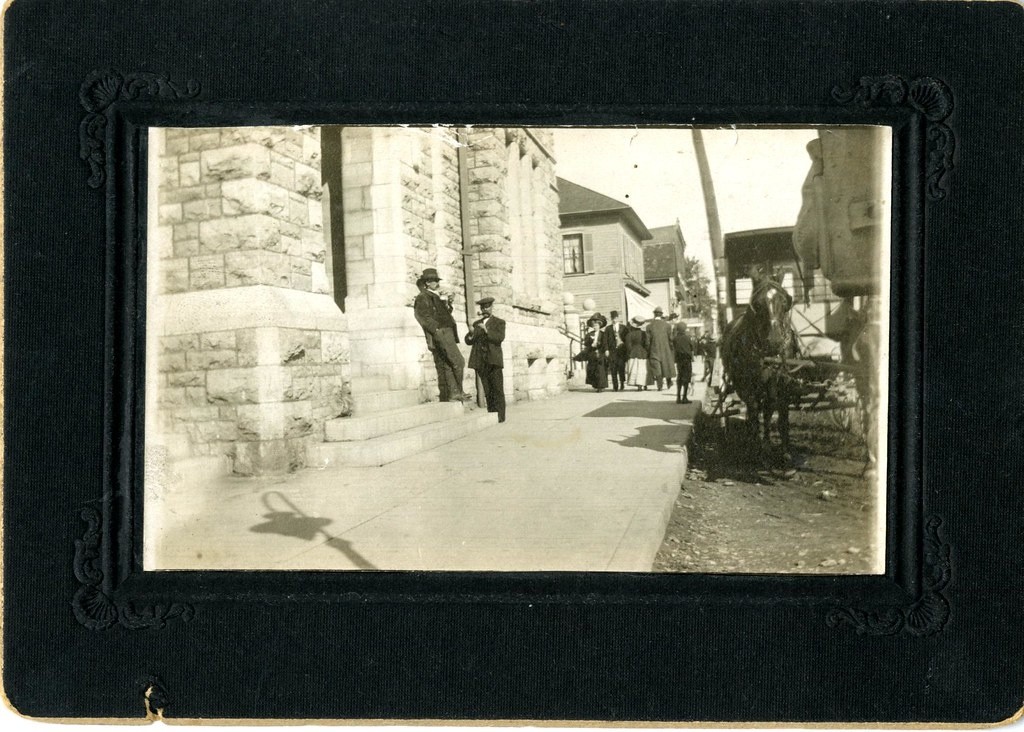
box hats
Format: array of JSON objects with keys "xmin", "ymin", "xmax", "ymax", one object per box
[
  {"xmin": 420, "ymin": 268, "xmax": 442, "ymax": 281},
  {"xmin": 630, "ymin": 316, "xmax": 645, "ymax": 328},
  {"xmin": 668, "ymin": 313, "xmax": 679, "ymax": 320},
  {"xmin": 652, "ymin": 306, "xmax": 663, "ymax": 312},
  {"xmin": 587, "ymin": 312, "xmax": 607, "ymax": 328},
  {"xmin": 476, "ymin": 297, "xmax": 494, "ymax": 308},
  {"xmin": 610, "ymin": 310, "xmax": 618, "ymax": 320}
]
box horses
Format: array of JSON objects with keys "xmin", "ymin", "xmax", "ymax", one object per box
[{"xmin": 723, "ymin": 263, "xmax": 797, "ymax": 451}]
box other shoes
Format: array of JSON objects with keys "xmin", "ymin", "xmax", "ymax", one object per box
[
  {"xmin": 668, "ymin": 381, "xmax": 673, "ymax": 388},
  {"xmin": 448, "ymin": 391, "xmax": 473, "ymax": 401},
  {"xmin": 597, "ymin": 387, "xmax": 605, "ymax": 392},
  {"xmin": 638, "ymin": 385, "xmax": 642, "ymax": 391},
  {"xmin": 497, "ymin": 410, "xmax": 505, "ymax": 423},
  {"xmin": 658, "ymin": 386, "xmax": 662, "ymax": 391},
  {"xmin": 676, "ymin": 399, "xmax": 682, "ymax": 403},
  {"xmin": 644, "ymin": 386, "xmax": 647, "ymax": 390},
  {"xmin": 614, "ymin": 382, "xmax": 618, "ymax": 391},
  {"xmin": 682, "ymin": 399, "xmax": 691, "ymax": 404},
  {"xmin": 621, "ymin": 383, "xmax": 624, "ymax": 389}
]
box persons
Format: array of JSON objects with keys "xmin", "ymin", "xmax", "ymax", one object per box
[
  {"xmin": 625, "ymin": 315, "xmax": 649, "ymax": 392},
  {"xmin": 668, "ymin": 313, "xmax": 694, "ymax": 404},
  {"xmin": 700, "ymin": 331, "xmax": 714, "ymax": 341},
  {"xmin": 464, "ymin": 297, "xmax": 507, "ymax": 424},
  {"xmin": 414, "ymin": 268, "xmax": 472, "ymax": 402},
  {"xmin": 572, "ymin": 312, "xmax": 607, "ymax": 393},
  {"xmin": 646, "ymin": 306, "xmax": 677, "ymax": 391},
  {"xmin": 603, "ymin": 310, "xmax": 629, "ymax": 391}
]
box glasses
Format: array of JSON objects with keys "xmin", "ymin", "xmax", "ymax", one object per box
[{"xmin": 430, "ymin": 279, "xmax": 439, "ymax": 282}]
